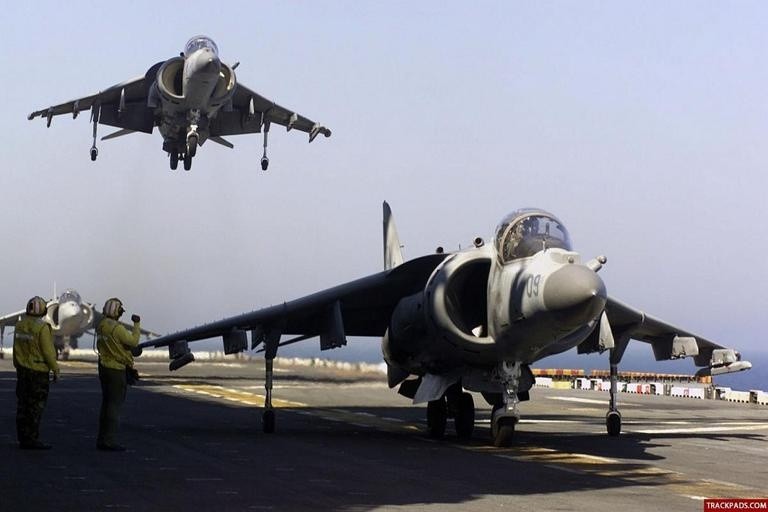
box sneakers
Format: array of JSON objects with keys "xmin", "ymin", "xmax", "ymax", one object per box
[
  {"xmin": 23, "ymin": 441, "xmax": 51, "ymax": 450},
  {"xmin": 95, "ymin": 439, "xmax": 124, "ymax": 450}
]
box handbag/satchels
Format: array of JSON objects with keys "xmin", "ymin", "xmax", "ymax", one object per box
[{"xmin": 125, "ymin": 364, "xmax": 139, "ymax": 386}]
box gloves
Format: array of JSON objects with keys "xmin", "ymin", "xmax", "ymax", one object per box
[{"xmin": 132, "ymin": 315, "xmax": 141, "ymax": 323}]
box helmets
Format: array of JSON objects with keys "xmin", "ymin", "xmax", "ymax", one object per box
[
  {"xmin": 105, "ymin": 297, "xmax": 124, "ymax": 320},
  {"xmin": 27, "ymin": 296, "xmax": 47, "ymax": 317}
]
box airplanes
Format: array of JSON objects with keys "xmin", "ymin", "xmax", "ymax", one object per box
[
  {"xmin": 138, "ymin": 199, "xmax": 752, "ymax": 448},
  {"xmin": 27, "ymin": 34, "xmax": 331, "ymax": 171},
  {"xmin": 0, "ymin": 290, "xmax": 161, "ymax": 360}
]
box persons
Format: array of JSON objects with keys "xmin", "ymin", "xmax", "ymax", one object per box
[
  {"xmin": 95, "ymin": 298, "xmax": 141, "ymax": 452},
  {"xmin": 11, "ymin": 295, "xmax": 60, "ymax": 450}
]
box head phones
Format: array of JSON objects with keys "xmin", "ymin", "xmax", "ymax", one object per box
[
  {"xmin": 25, "ymin": 307, "xmax": 47, "ymax": 317},
  {"xmin": 103, "ymin": 297, "xmax": 125, "ymax": 316}
]
